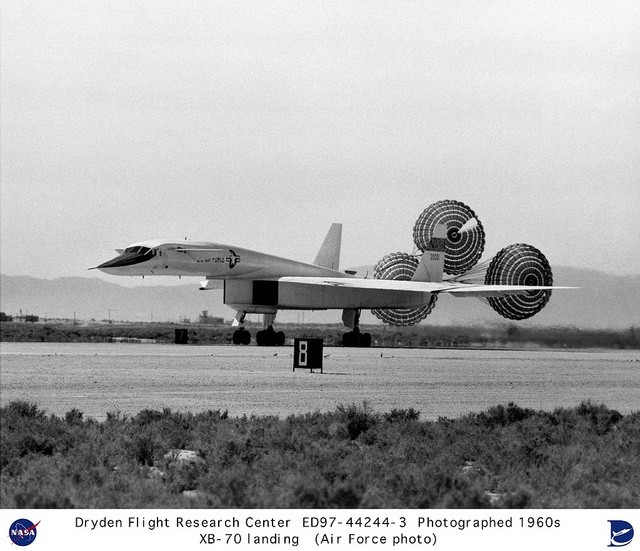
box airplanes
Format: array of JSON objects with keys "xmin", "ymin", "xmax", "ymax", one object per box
[{"xmin": 86, "ymin": 200, "xmax": 586, "ymax": 348}]
